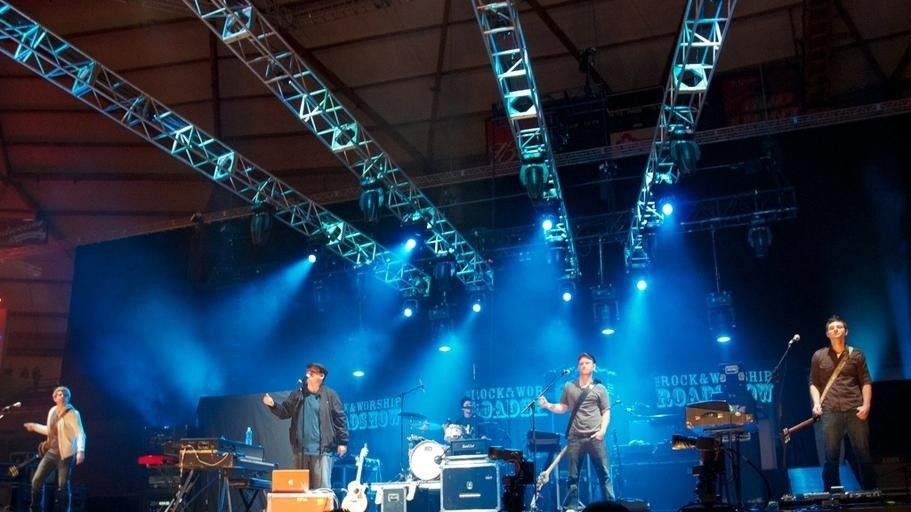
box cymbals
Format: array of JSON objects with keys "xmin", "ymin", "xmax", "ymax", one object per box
[{"xmin": 398, "ymin": 412, "xmax": 425, "ymax": 419}]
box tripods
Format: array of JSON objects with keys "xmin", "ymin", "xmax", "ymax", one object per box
[{"xmin": 386, "ymin": 385, "xmax": 421, "ymax": 482}]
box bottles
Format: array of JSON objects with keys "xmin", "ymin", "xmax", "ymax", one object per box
[{"xmin": 245, "ymin": 426, "xmax": 253, "ymax": 447}]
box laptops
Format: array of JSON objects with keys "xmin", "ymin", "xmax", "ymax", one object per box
[{"xmin": 269, "ymin": 469, "xmax": 309, "ymax": 493}]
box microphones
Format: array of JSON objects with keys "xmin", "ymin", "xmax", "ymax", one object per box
[
  {"xmin": 472, "ymin": 360, "xmax": 476, "ymax": 382},
  {"xmin": 297, "ymin": 379, "xmax": 303, "ymax": 392},
  {"xmin": 788, "ymin": 334, "xmax": 801, "ymax": 346},
  {"xmin": 418, "ymin": 377, "xmax": 425, "ymax": 393},
  {"xmin": 563, "ymin": 369, "xmax": 570, "ymax": 374},
  {"xmin": 2, "ymin": 401, "xmax": 22, "ymax": 411}
]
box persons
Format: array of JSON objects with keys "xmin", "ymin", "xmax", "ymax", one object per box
[
  {"xmin": 262, "ymin": 361, "xmax": 350, "ymax": 490},
  {"xmin": 444, "ymin": 397, "xmax": 484, "ymax": 439},
  {"xmin": 24, "ymin": 386, "xmax": 88, "ymax": 511},
  {"xmin": 808, "ymin": 314, "xmax": 884, "ymax": 495},
  {"xmin": 539, "ymin": 351, "xmax": 617, "ymax": 512}
]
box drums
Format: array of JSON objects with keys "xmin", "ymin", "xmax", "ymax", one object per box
[
  {"xmin": 443, "ymin": 424, "xmax": 465, "ymax": 442},
  {"xmin": 502, "ymin": 476, "xmax": 515, "ymax": 485},
  {"xmin": 409, "ymin": 441, "xmax": 448, "ymax": 480},
  {"xmin": 488, "ymin": 446, "xmax": 522, "ymax": 461},
  {"xmin": 515, "ymin": 460, "xmax": 533, "ymax": 484}
]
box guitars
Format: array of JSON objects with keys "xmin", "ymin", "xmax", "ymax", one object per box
[{"xmin": 341, "ymin": 448, "xmax": 369, "ymax": 512}]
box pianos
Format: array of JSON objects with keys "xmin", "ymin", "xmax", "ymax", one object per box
[
  {"xmin": 178, "ymin": 438, "xmax": 279, "ymax": 473},
  {"xmin": 526, "ymin": 429, "xmax": 567, "ymax": 452},
  {"xmin": 685, "ymin": 401, "xmax": 753, "ymax": 429}
]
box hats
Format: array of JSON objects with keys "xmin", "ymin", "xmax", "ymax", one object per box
[
  {"xmin": 307, "ymin": 363, "xmax": 328, "ymax": 375},
  {"xmin": 578, "ymin": 352, "xmax": 595, "ymax": 363}
]
